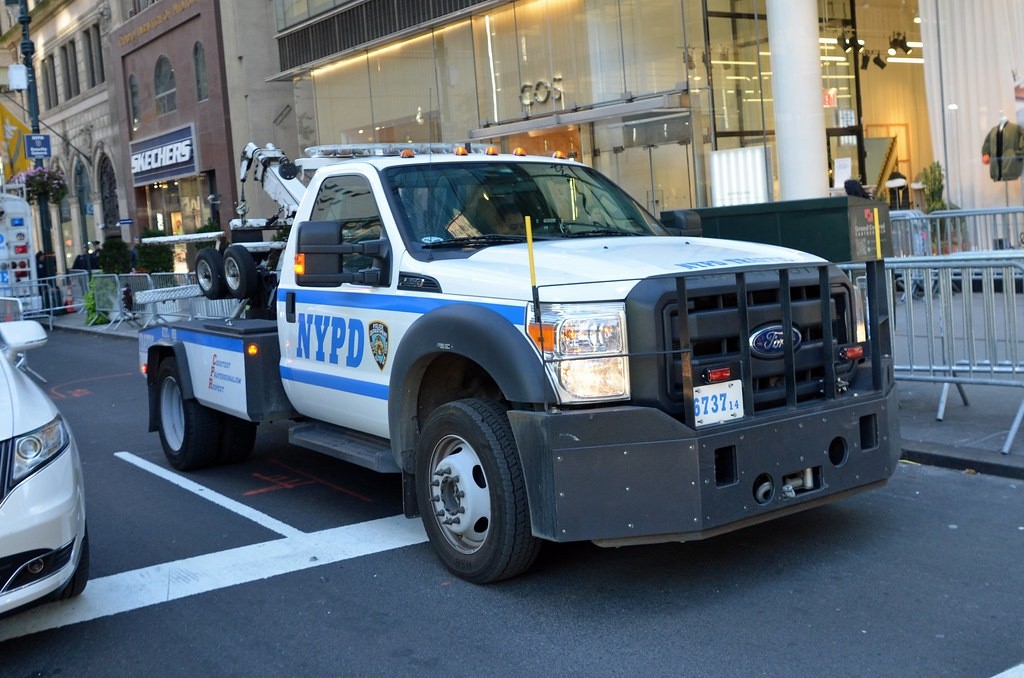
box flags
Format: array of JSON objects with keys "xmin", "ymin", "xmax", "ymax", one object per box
[{"xmin": 1, "ymin": 97, "xmax": 45, "ymax": 185}]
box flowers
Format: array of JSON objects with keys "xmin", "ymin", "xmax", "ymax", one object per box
[{"xmin": 25, "ymin": 167, "xmax": 69, "ymax": 204}]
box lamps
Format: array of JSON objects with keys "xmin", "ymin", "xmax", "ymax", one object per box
[
  {"xmin": 888, "ymin": 37, "xmax": 900, "ymax": 55},
  {"xmin": 154, "ymin": 184, "xmax": 158, "ymax": 189},
  {"xmin": 159, "ymin": 183, "xmax": 162, "ymax": 187},
  {"xmin": 682, "ymin": 49, "xmax": 696, "ymax": 69},
  {"xmin": 719, "ymin": 49, "xmax": 731, "ymax": 70},
  {"xmin": 848, "ymin": 34, "xmax": 864, "ymax": 53},
  {"xmin": 837, "ymin": 33, "xmax": 851, "ymax": 53},
  {"xmin": 861, "ymin": 52, "xmax": 870, "ymax": 69},
  {"xmin": 701, "ymin": 49, "xmax": 715, "ymax": 69},
  {"xmin": 872, "ymin": 52, "xmax": 887, "ymax": 70},
  {"xmin": 899, "ymin": 35, "xmax": 912, "ymax": 55},
  {"xmin": 162, "ymin": 183, "xmax": 168, "ymax": 188},
  {"xmin": 174, "ymin": 181, "xmax": 179, "ymax": 185}
]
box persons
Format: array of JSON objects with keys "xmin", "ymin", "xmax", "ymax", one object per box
[
  {"xmin": 982, "ymin": 114, "xmax": 1024, "ymax": 184},
  {"xmin": 493, "ymin": 201, "xmax": 528, "ymax": 237},
  {"xmin": 34, "ymin": 250, "xmax": 70, "ymax": 316},
  {"xmin": 87, "ymin": 239, "xmax": 106, "ymax": 268}
]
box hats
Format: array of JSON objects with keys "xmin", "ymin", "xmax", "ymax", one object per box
[
  {"xmin": 83, "ymin": 245, "xmax": 89, "ymax": 250},
  {"xmin": 134, "ymin": 236, "xmax": 139, "ymax": 239},
  {"xmin": 91, "ymin": 241, "xmax": 100, "ymax": 245}
]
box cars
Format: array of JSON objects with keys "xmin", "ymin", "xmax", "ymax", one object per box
[{"xmin": 0, "ymin": 321, "xmax": 90, "ymax": 615}]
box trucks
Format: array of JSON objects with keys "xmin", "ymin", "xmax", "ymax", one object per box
[
  {"xmin": 134, "ymin": 141, "xmax": 903, "ymax": 587},
  {"xmin": 0, "ymin": 194, "xmax": 43, "ymax": 316}
]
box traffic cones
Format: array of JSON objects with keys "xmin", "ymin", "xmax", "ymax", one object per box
[{"xmin": 65, "ymin": 286, "xmax": 77, "ymax": 313}]
